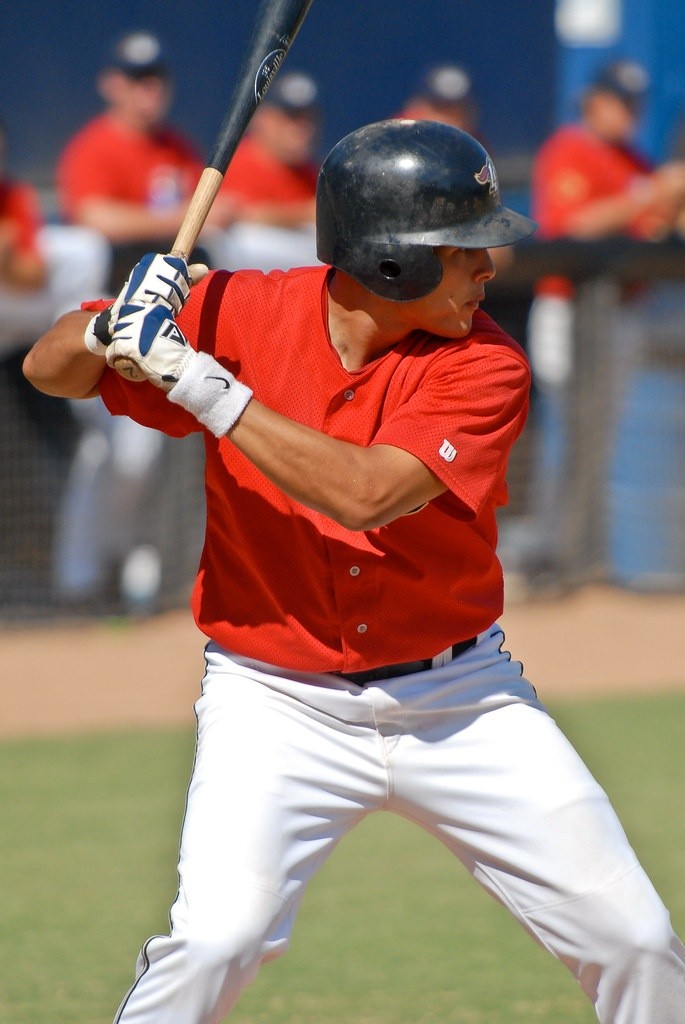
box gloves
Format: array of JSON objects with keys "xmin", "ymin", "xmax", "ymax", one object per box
[
  {"xmin": 102, "ymin": 301, "xmax": 202, "ymax": 392},
  {"xmin": 83, "ymin": 253, "xmax": 210, "ymax": 359}
]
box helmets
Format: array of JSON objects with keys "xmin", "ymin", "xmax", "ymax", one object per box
[{"xmin": 313, "ymin": 118, "xmax": 537, "ymax": 304}]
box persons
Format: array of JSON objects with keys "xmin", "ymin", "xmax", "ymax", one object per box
[
  {"xmin": 23, "ymin": 120, "xmax": 685, "ymax": 1024},
  {"xmin": 0, "ymin": 33, "xmax": 685, "ymax": 618}
]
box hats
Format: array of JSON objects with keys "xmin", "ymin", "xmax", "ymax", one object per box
[
  {"xmin": 598, "ymin": 63, "xmax": 655, "ymax": 120},
  {"xmin": 415, "ymin": 60, "xmax": 497, "ymax": 115},
  {"xmin": 267, "ymin": 63, "xmax": 328, "ymax": 117},
  {"xmin": 89, "ymin": 31, "xmax": 184, "ymax": 113}
]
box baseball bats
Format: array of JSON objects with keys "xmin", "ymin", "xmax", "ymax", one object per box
[{"xmin": 109, "ymin": 0, "xmax": 318, "ymax": 389}]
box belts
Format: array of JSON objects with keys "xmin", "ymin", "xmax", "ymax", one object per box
[{"xmin": 329, "ymin": 632, "xmax": 492, "ymax": 681}]
